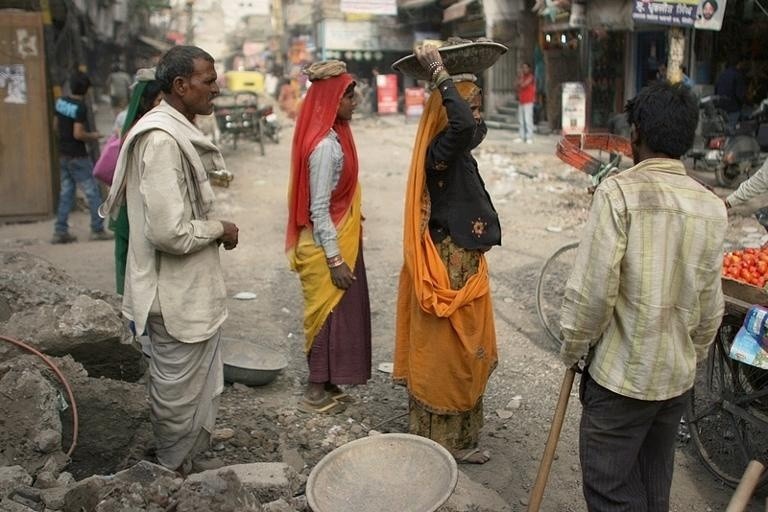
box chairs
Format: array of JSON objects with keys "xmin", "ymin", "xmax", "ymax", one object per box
[{"xmin": 212, "ymin": 90, "xmax": 280, "ymax": 158}]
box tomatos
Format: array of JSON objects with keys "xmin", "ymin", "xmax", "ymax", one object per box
[{"xmin": 723, "ymin": 244, "xmax": 768, "ymax": 288}]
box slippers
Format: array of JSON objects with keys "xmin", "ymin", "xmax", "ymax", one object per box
[
  {"xmin": 295, "ymin": 394, "xmax": 348, "ymax": 416},
  {"xmin": 328, "ymin": 391, "xmax": 360, "ymax": 407},
  {"xmin": 454, "ymin": 448, "xmax": 490, "ymax": 465}
]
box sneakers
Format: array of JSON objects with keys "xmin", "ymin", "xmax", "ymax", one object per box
[
  {"xmin": 527, "ymin": 139, "xmax": 533, "ymax": 144},
  {"xmin": 52, "ymin": 233, "xmax": 78, "ymax": 244},
  {"xmin": 89, "ymin": 231, "xmax": 115, "ymax": 240},
  {"xmin": 513, "ymin": 138, "xmax": 523, "ymax": 143}
]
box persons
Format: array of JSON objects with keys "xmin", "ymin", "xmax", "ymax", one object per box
[
  {"xmin": 392, "ymin": 39, "xmax": 503, "ymax": 466},
  {"xmin": 512, "ymin": 62, "xmax": 537, "ymax": 145},
  {"xmin": 559, "ymin": 81, "xmax": 728, "ymax": 512},
  {"xmin": 714, "ymin": 54, "xmax": 745, "ymax": 129},
  {"xmin": 724, "ymin": 156, "xmax": 768, "ymax": 208},
  {"xmin": 284, "ymin": 58, "xmax": 372, "ymax": 416},
  {"xmin": 693, "ymin": 0, "xmax": 721, "ymax": 31}
]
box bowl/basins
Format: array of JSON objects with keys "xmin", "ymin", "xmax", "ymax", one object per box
[{"xmin": 215, "ymin": 337, "xmax": 289, "ymax": 388}]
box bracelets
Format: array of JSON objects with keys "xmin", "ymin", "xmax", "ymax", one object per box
[
  {"xmin": 426, "ymin": 62, "xmax": 446, "ymax": 82},
  {"xmin": 328, "ymin": 256, "xmax": 344, "ymax": 268},
  {"xmin": 726, "ymin": 199, "xmax": 730, "ymax": 207}
]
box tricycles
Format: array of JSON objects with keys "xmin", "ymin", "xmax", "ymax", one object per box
[
  {"xmin": 211, "ymin": 103, "xmax": 267, "ymax": 157},
  {"xmin": 534, "ymin": 154, "xmax": 768, "ymax": 497}
]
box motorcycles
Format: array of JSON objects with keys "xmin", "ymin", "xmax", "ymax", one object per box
[
  {"xmin": 239, "ymin": 97, "xmax": 283, "ymax": 145},
  {"xmin": 693, "ymin": 93, "xmax": 767, "ymax": 186}
]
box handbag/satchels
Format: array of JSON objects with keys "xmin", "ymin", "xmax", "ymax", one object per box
[{"xmin": 92, "ymin": 132, "xmax": 122, "ymax": 186}]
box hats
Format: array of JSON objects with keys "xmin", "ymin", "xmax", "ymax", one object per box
[{"xmin": 135, "ymin": 66, "xmax": 158, "ymax": 81}]
